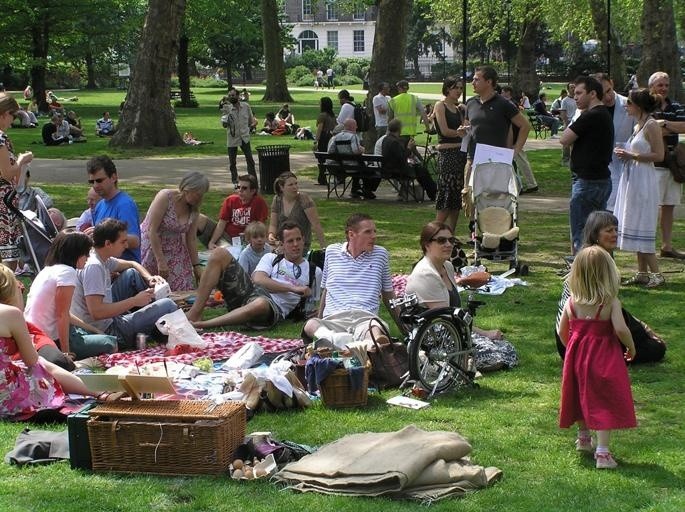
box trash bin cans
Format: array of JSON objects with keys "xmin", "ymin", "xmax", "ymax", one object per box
[{"xmin": 255, "ymin": 145, "xmax": 292, "ymax": 196}]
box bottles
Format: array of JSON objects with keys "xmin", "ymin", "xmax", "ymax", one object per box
[
  {"xmin": 136, "ymin": 333, "xmax": 145, "ymax": 350},
  {"xmin": 307, "ymin": 346, "xmax": 314, "ymax": 358}
]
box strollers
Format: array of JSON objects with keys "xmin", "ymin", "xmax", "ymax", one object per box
[
  {"xmin": 469, "ymin": 159, "xmax": 528, "ymax": 276},
  {"xmin": 4, "ymin": 189, "xmax": 60, "ymax": 273}
]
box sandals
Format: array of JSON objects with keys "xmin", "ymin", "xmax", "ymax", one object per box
[
  {"xmin": 97, "ymin": 392, "xmax": 126, "ymax": 402},
  {"xmin": 645, "ymin": 273, "xmax": 665, "ymax": 288},
  {"xmin": 622, "ymin": 271, "xmax": 650, "ymax": 285}
]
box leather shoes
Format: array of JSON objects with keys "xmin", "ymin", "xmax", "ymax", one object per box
[
  {"xmin": 525, "ymin": 187, "xmax": 540, "ymax": 194},
  {"xmin": 520, "ymin": 189, "xmax": 526, "ymax": 196},
  {"xmin": 661, "ymin": 248, "xmax": 685, "ymax": 259}
]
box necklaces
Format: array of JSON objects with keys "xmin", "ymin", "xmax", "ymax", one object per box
[{"xmin": 440, "ymin": 273, "xmax": 444, "ymax": 278}]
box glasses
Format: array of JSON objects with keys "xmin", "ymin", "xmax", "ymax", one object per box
[
  {"xmin": 11, "ymin": 113, "xmax": 17, "ymax": 120},
  {"xmin": 85, "ymin": 249, "xmax": 90, "ymax": 258},
  {"xmin": 237, "ymin": 185, "xmax": 250, "ymax": 190},
  {"xmin": 87, "ymin": 176, "xmax": 108, "ymax": 184},
  {"xmin": 627, "ymin": 99, "xmax": 634, "ymax": 105},
  {"xmin": 430, "ymin": 236, "xmax": 455, "ymax": 244},
  {"xmin": 227, "ymin": 95, "xmax": 236, "ymax": 98}
]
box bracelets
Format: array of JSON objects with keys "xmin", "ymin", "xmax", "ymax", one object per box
[
  {"xmin": 633, "ymin": 154, "xmax": 639, "ymax": 161},
  {"xmin": 193, "ymin": 263, "xmax": 204, "ymax": 266}
]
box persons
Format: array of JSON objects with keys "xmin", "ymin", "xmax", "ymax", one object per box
[
  {"xmin": 57, "ymin": 113, "xmax": 70, "ymax": 138},
  {"xmin": 336, "ymin": 90, "xmax": 357, "ymax": 132},
  {"xmin": 238, "ymin": 222, "xmax": 272, "ymax": 276},
  {"xmin": 381, "ymin": 119, "xmax": 437, "ymax": 201},
  {"xmin": 302, "ymin": 213, "xmax": 413, "ymax": 351},
  {"xmin": 317, "ymin": 67, "xmax": 324, "ymax": 90},
  {"xmin": 21, "ymin": 229, "xmax": 118, "ymax": 361},
  {"xmin": 64, "ymin": 111, "xmax": 87, "ymax": 142},
  {"xmin": 46, "ymin": 208, "xmax": 66, "ymax": 230},
  {"xmin": 221, "ymin": 87, "xmax": 258, "ymax": 193},
  {"xmin": 614, "ymin": 88, "xmax": 665, "ymax": 288},
  {"xmin": 276, "ymin": 104, "xmax": 294, "ymax": 124},
  {"xmin": 534, "ymin": 93, "xmax": 562, "ymax": 139},
  {"xmin": 561, "ymin": 75, "xmax": 614, "ymax": 257},
  {"xmin": 375, "ymin": 128, "xmax": 424, "ymax": 202},
  {"xmin": 518, "ymin": 92, "xmax": 531, "ymax": 109},
  {"xmin": 140, "ymin": 172, "xmax": 210, "ymax": 291},
  {"xmin": 27, "ymin": 97, "xmax": 38, "ymax": 115},
  {"xmin": 0, "ymin": 91, "xmax": 33, "ymax": 274},
  {"xmin": 594, "ymin": 73, "xmax": 635, "ymax": 212},
  {"xmin": 261, "ymin": 112, "xmax": 279, "ymax": 134},
  {"xmin": 556, "ymin": 210, "xmax": 667, "ymax": 363},
  {"xmin": 327, "ymin": 119, "xmax": 376, "ymax": 199},
  {"xmin": 326, "ymin": 66, "xmax": 335, "ymax": 89},
  {"xmin": 461, "ymin": 66, "xmax": 530, "ymax": 189},
  {"xmin": 373, "ymin": 82, "xmax": 392, "ymax": 139},
  {"xmin": 432, "ymin": 75, "xmax": 467, "ymax": 235},
  {"xmin": 268, "ymin": 172, "xmax": 325, "ymax": 249},
  {"xmin": 42, "ymin": 114, "xmax": 69, "ymax": 145},
  {"xmin": 419, "ymin": 105, "xmax": 434, "ymax": 123},
  {"xmin": 558, "ymin": 245, "xmax": 636, "ymax": 469},
  {"xmin": 240, "ymin": 89, "xmax": 248, "ymax": 102},
  {"xmin": 118, "ymin": 96, "xmax": 126, "ymax": 114},
  {"xmin": 96, "ymin": 112, "xmax": 114, "ymax": 138},
  {"xmin": 364, "ymin": 68, "xmax": 370, "ymax": 89},
  {"xmin": 185, "ymin": 220, "xmax": 322, "ymax": 330},
  {"xmin": 387, "ymin": 80, "xmax": 430, "ymax": 149},
  {"xmin": 23, "ymin": 86, "xmax": 33, "ymax": 100},
  {"xmin": 48, "ymin": 98, "xmax": 63, "ymax": 117},
  {"xmin": 46, "ymin": 91, "xmax": 63, "ymax": 103},
  {"xmin": 82, "ymin": 155, "xmax": 141, "ymax": 264},
  {"xmin": 75, "ymin": 187, "xmax": 101, "ymax": 232},
  {"xmin": 219, "ymin": 96, "xmax": 227, "ymax": 107},
  {"xmin": 197, "ymin": 175, "xmax": 268, "ymax": 250},
  {"xmin": 312, "ymin": 97, "xmax": 345, "ymax": 185},
  {"xmin": 69, "ymin": 218, "xmax": 177, "ymax": 350},
  {"xmin": 249, "ymin": 112, "xmax": 258, "ymax": 134},
  {"xmin": 405, "ymin": 221, "xmax": 519, "ymax": 372},
  {"xmin": 0, "ymin": 264, "xmax": 127, "ymax": 421},
  {"xmin": 648, "ymin": 72, "xmax": 685, "ymax": 259},
  {"xmin": 69, "ymin": 96, "xmax": 78, "ymax": 101},
  {"xmin": 0, "ymin": 83, "xmax": 38, "ymax": 128},
  {"xmin": 550, "ymin": 90, "xmax": 567, "ymax": 115},
  {"xmin": 560, "ymin": 83, "xmax": 577, "ymax": 163},
  {"xmin": 501, "ymin": 87, "xmax": 538, "ymax": 195}
]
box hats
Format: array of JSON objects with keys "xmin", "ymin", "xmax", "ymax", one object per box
[{"xmin": 396, "ymin": 80, "xmax": 408, "ymax": 88}]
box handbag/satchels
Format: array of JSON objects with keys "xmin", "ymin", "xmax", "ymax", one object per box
[
  {"xmin": 256, "ymin": 368, "xmax": 312, "ymax": 413},
  {"xmin": 367, "ymin": 317, "xmax": 410, "ymax": 388},
  {"xmin": 218, "ymin": 98, "xmax": 236, "ymax": 138},
  {"xmin": 456, "ymin": 106, "xmax": 465, "ymax": 127},
  {"xmin": 653, "ymin": 111, "xmax": 685, "ymax": 182},
  {"xmin": 457, "ymin": 271, "xmax": 491, "ymax": 287},
  {"xmin": 269, "ymin": 347, "xmax": 307, "ymax": 389}
]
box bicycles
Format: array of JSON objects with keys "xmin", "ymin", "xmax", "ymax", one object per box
[{"xmin": 411, "ymin": 129, "xmax": 439, "ymax": 192}]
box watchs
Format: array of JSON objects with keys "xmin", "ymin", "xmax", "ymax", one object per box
[
  {"xmin": 314, "ymin": 141, "xmax": 318, "ymax": 145},
  {"xmin": 664, "ymin": 120, "xmax": 667, "ymax": 128}
]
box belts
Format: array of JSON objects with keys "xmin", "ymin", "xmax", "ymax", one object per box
[{"xmin": 572, "ymin": 172, "xmax": 610, "ymax": 180}]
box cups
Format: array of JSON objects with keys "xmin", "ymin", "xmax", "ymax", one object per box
[
  {"xmin": 232, "ymin": 236, "xmax": 241, "ymax": 246},
  {"xmin": 616, "ymin": 141, "xmax": 625, "ymax": 149}
]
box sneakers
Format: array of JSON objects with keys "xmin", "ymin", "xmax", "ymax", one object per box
[
  {"xmin": 551, "ymin": 134, "xmax": 560, "ymax": 138},
  {"xmin": 576, "ymin": 436, "xmax": 593, "ymax": 451},
  {"xmin": 233, "ymin": 184, "xmax": 238, "ymax": 188},
  {"xmin": 594, "ymin": 453, "xmax": 618, "ymax": 469}
]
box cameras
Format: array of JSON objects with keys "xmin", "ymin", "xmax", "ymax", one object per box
[{"xmin": 221, "ymin": 96, "xmax": 239, "ymax": 104}]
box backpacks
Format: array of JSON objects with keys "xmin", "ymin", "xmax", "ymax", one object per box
[{"xmin": 347, "ymin": 102, "xmax": 371, "ymax": 132}]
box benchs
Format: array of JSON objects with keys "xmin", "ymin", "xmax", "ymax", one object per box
[
  {"xmin": 313, "ymin": 152, "xmax": 422, "ymax": 202},
  {"xmin": 527, "ymin": 109, "xmax": 568, "ymax": 139},
  {"xmin": 171, "ymin": 91, "xmax": 195, "ymax": 99}
]
